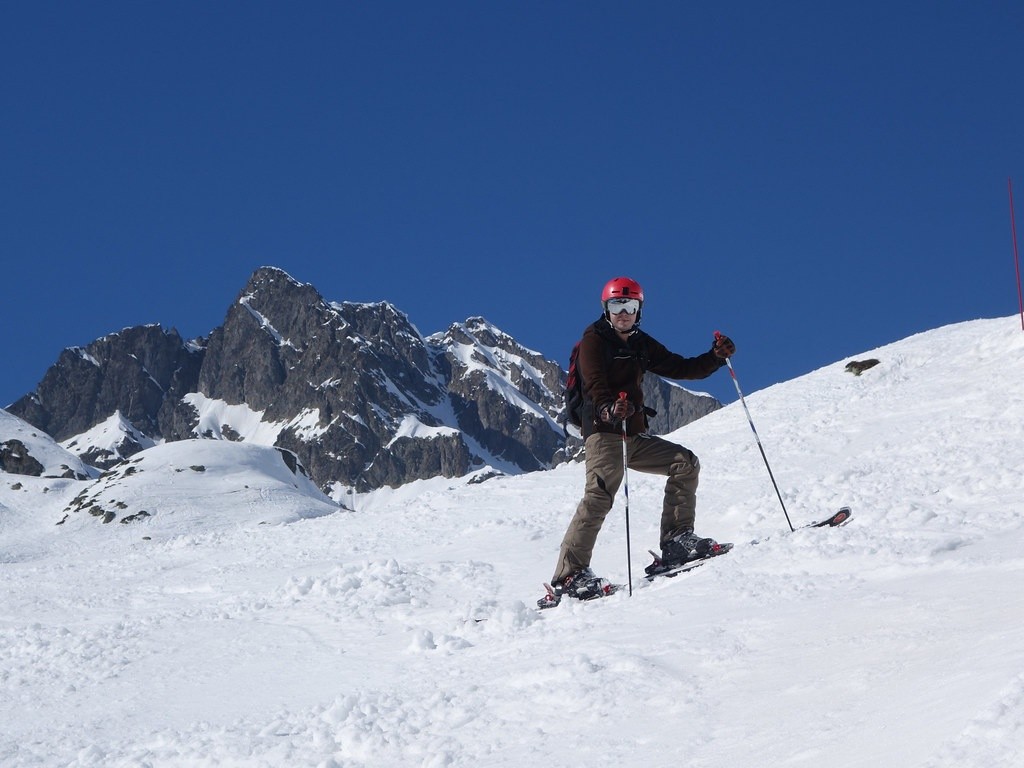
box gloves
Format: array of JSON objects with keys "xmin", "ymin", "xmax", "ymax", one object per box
[
  {"xmin": 712, "ymin": 335, "xmax": 735, "ymax": 359},
  {"xmin": 609, "ymin": 397, "xmax": 635, "ymax": 418}
]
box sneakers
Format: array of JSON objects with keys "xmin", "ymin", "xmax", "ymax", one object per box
[
  {"xmin": 555, "ymin": 568, "xmax": 611, "ymax": 599},
  {"xmin": 661, "ymin": 532, "xmax": 717, "ymax": 566}
]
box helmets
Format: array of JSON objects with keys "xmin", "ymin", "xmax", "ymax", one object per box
[{"xmin": 602, "ymin": 277, "xmax": 644, "ymax": 303}]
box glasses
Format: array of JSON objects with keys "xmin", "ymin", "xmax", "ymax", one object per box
[{"xmin": 601, "ymin": 299, "xmax": 640, "ymax": 314}]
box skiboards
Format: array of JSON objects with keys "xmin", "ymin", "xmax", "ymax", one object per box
[{"xmin": 461, "ymin": 507, "xmax": 855, "ymax": 627}]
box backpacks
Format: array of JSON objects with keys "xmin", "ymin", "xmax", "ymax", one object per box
[{"xmin": 563, "ymin": 329, "xmax": 657, "ymax": 438}]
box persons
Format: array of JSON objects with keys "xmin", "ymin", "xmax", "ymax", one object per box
[{"xmin": 551, "ymin": 277, "xmax": 735, "ymax": 599}]
